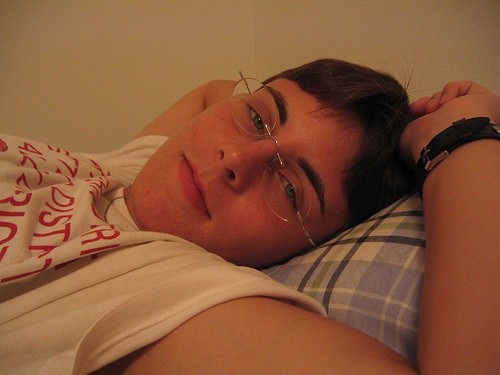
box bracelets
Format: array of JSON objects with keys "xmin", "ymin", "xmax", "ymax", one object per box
[{"xmin": 414, "ymin": 116, "xmax": 500, "ymax": 187}]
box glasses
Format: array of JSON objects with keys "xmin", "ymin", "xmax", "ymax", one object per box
[{"xmin": 229, "ymin": 70, "xmax": 316, "ymax": 247}]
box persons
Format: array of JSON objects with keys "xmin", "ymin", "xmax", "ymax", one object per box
[{"xmin": 0, "ymin": 55, "xmax": 500, "ymax": 374}]
